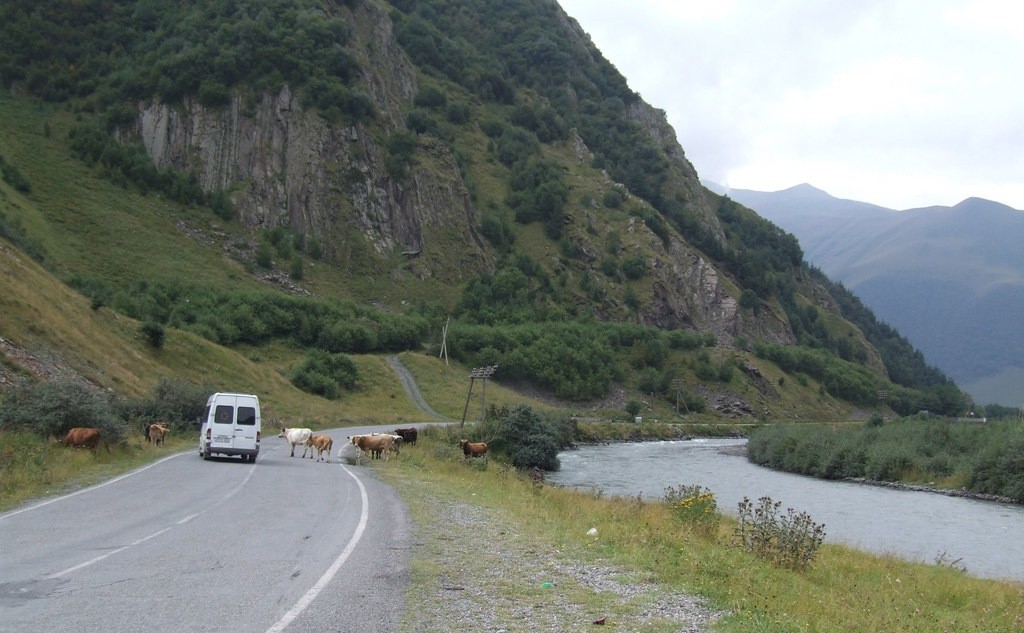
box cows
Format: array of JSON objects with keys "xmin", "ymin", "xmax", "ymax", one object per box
[
  {"xmin": 347, "ymin": 426, "xmax": 417, "ymax": 460},
  {"xmin": 55, "ymin": 427, "xmax": 109, "ymax": 454},
  {"xmin": 278, "ymin": 428, "xmax": 334, "ymax": 463},
  {"xmin": 461, "ymin": 439, "xmax": 488, "ymax": 460},
  {"xmin": 143, "ymin": 420, "xmax": 170, "ymax": 446}
]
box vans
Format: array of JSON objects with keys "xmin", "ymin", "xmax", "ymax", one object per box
[{"xmin": 196, "ymin": 392, "xmax": 261, "ymax": 463}]
